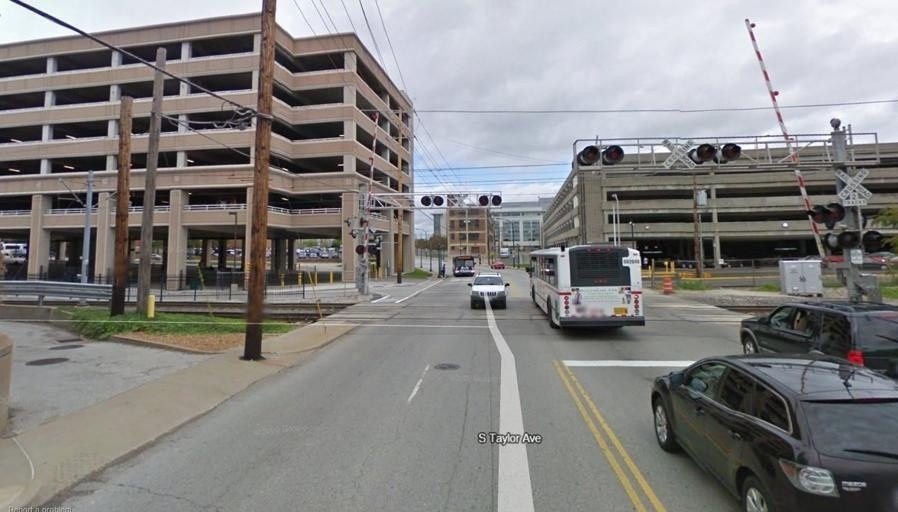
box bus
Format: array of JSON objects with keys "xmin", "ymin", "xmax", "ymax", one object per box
[
  {"xmin": 525, "ymin": 243, "xmax": 646, "ymax": 333},
  {"xmin": 452, "ymin": 255, "xmax": 476, "ymax": 277}
]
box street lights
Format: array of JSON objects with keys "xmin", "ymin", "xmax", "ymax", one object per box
[
  {"xmin": 496, "ymin": 217, "xmax": 515, "ymax": 267},
  {"xmin": 630, "ymin": 221, "xmax": 635, "ymax": 249},
  {"xmin": 229, "ymin": 211, "xmax": 238, "ymax": 289},
  {"xmin": 611, "ymin": 193, "xmax": 621, "ymax": 246},
  {"xmin": 415, "ymin": 226, "xmax": 429, "ymax": 260}
]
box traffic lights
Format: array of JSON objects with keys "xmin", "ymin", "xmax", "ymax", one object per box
[
  {"xmin": 689, "ymin": 144, "xmax": 716, "ymax": 165},
  {"xmin": 602, "ymin": 144, "xmax": 625, "ymax": 165},
  {"xmin": 421, "ymin": 196, "xmax": 432, "ymax": 206},
  {"xmin": 808, "ymin": 203, "xmax": 843, "ymax": 227},
  {"xmin": 492, "ymin": 196, "xmax": 501, "ymax": 205},
  {"xmin": 824, "ymin": 230, "xmax": 885, "ymax": 251},
  {"xmin": 714, "ymin": 144, "xmax": 740, "ymax": 164},
  {"xmin": 433, "ymin": 196, "xmax": 444, "ymax": 206},
  {"xmin": 367, "ymin": 245, "xmax": 377, "ymax": 254},
  {"xmin": 356, "ymin": 245, "xmax": 365, "ymax": 254},
  {"xmin": 479, "ymin": 196, "xmax": 488, "ymax": 205},
  {"xmin": 577, "ymin": 146, "xmax": 600, "ymax": 166}
]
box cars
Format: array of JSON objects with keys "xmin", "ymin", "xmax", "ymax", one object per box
[
  {"xmin": 189, "ymin": 246, "xmax": 339, "ymax": 259},
  {"xmin": 647, "ymin": 351, "xmax": 898, "ymax": 511},
  {"xmin": 491, "ymin": 261, "xmax": 506, "ymax": 269},
  {"xmin": 804, "ymin": 250, "xmax": 898, "ymax": 270},
  {"xmin": 134, "ymin": 253, "xmax": 163, "ymax": 265}
]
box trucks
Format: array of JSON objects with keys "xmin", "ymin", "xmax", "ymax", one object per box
[{"xmin": 499, "ymin": 247, "xmax": 509, "ymax": 258}]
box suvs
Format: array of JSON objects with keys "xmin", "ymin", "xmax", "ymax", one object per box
[
  {"xmin": 737, "ymin": 298, "xmax": 898, "ymax": 379},
  {"xmin": 468, "ymin": 272, "xmax": 511, "ymax": 310}
]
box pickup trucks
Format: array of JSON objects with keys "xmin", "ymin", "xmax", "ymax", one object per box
[{"xmin": 670, "ymin": 255, "xmax": 733, "ymax": 269}]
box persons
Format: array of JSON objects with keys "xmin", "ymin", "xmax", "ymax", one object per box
[{"xmin": 443, "ymin": 264, "xmax": 445, "ymax": 278}]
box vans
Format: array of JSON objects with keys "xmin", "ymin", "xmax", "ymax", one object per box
[{"xmin": 1, "ymin": 242, "xmax": 28, "ymax": 257}]
